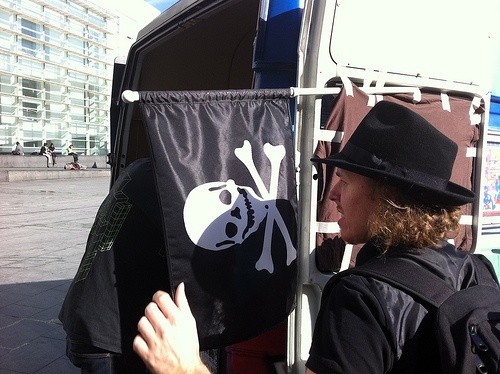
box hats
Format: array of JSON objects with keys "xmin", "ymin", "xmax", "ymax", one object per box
[{"xmin": 310, "ymin": 99, "xmax": 479, "ymax": 204}]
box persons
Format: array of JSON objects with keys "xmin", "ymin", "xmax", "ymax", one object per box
[
  {"xmin": 133, "ymin": 100, "xmax": 499, "ymax": 374},
  {"xmin": 49, "ymin": 143, "xmax": 57, "ymax": 166},
  {"xmin": 67, "ymin": 144, "xmax": 79, "ymax": 164},
  {"xmin": 40, "ymin": 142, "xmax": 51, "ymax": 169},
  {"xmin": 63, "ymin": 162, "xmax": 88, "ymax": 170},
  {"xmin": 12, "ymin": 142, "xmax": 24, "ymax": 155}
]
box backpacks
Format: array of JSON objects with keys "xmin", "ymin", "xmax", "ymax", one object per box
[{"xmin": 349, "ymin": 252, "xmax": 500, "ymax": 374}]
